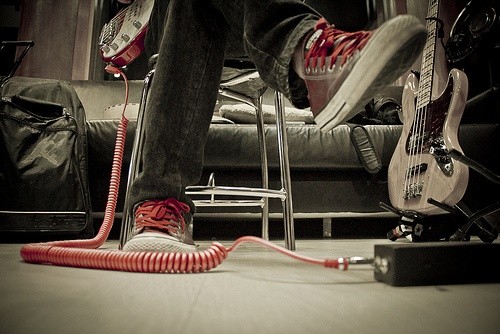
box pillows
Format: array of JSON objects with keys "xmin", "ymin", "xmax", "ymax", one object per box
[{"xmin": 218, "ymin": 104, "xmax": 315, "ymax": 125}]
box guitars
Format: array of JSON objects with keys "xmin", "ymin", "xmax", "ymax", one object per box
[
  {"xmin": 385, "ymin": 0, "xmax": 471, "ymax": 219},
  {"xmin": 95, "ymin": 0, "xmax": 157, "ymax": 70}
]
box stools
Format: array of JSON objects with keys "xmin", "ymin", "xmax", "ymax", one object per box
[{"xmin": 117, "ymin": 53, "xmax": 297, "ymax": 251}]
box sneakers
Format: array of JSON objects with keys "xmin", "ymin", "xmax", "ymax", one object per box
[
  {"xmin": 123, "ymin": 200, "xmax": 197, "ymax": 253},
  {"xmin": 302, "ymin": 14, "xmax": 428, "ymax": 134}
]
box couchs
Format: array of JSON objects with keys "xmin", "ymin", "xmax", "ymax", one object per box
[{"xmin": 71, "ymin": 80, "xmax": 500, "ymax": 239}]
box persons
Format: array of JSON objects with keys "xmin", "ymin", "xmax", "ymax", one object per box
[{"xmin": 108, "ymin": 0, "xmax": 429, "ymax": 253}]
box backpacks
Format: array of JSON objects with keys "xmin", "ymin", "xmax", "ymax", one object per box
[{"xmin": 0, "ymin": 74, "xmax": 94, "ymax": 241}]
